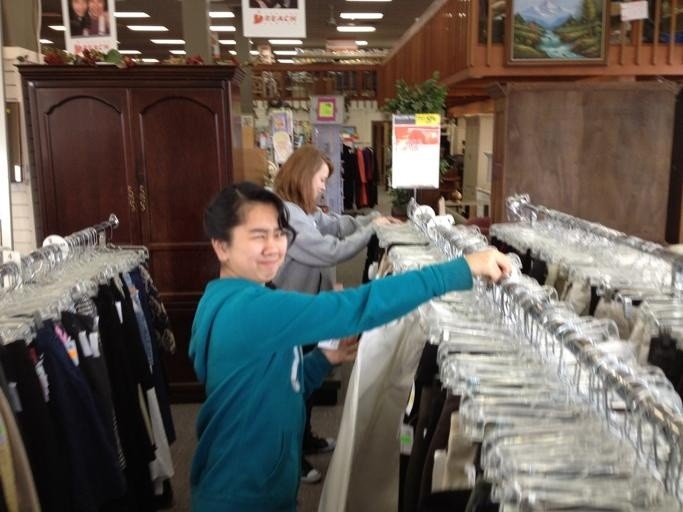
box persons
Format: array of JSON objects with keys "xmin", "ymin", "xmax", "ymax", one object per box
[
  {"xmin": 270, "ymin": 145, "xmax": 402, "ymax": 483},
  {"xmin": 186, "ymin": 183, "xmax": 510, "ymax": 511},
  {"xmin": 68, "ymin": 0, "xmax": 109, "ymax": 38}
]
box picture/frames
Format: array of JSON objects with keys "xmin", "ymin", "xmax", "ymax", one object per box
[{"xmin": 502, "ymin": 0, "xmax": 612, "ymax": 69}]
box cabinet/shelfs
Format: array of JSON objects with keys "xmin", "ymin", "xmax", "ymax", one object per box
[
  {"xmin": 462, "ymin": 111, "xmax": 495, "ymax": 217},
  {"xmin": 11, "ymin": 63, "xmax": 240, "ymax": 336}
]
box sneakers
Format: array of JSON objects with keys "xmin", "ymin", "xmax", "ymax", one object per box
[
  {"xmin": 300, "ymin": 459, "xmax": 322, "ymax": 484},
  {"xmin": 307, "ymin": 436, "xmax": 335, "ymax": 454}
]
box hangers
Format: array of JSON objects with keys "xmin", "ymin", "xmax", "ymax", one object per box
[{"xmin": 0, "ymin": 219, "xmax": 151, "ymax": 347}]
